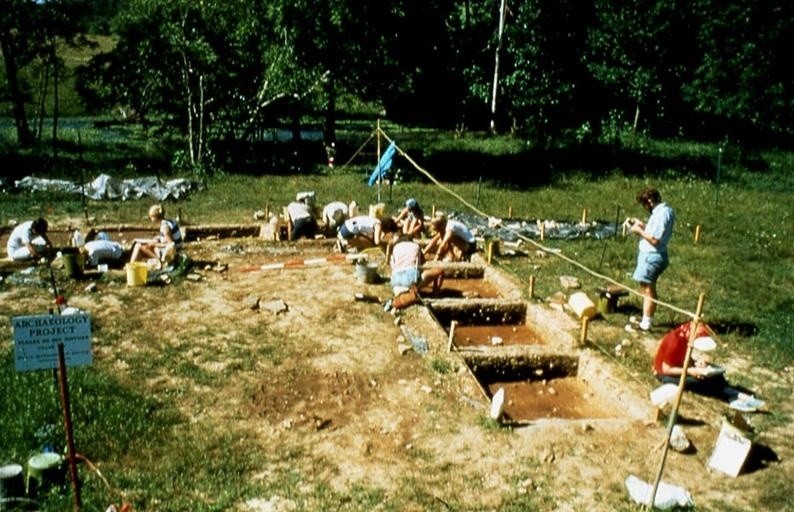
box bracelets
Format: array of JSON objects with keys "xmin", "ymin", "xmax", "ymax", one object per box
[{"xmin": 435, "ymin": 252, "xmax": 443, "ymax": 257}]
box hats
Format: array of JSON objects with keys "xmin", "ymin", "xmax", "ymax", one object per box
[
  {"xmin": 406, "ymin": 197, "xmax": 418, "ymax": 211},
  {"xmin": 686, "ymin": 322, "xmax": 717, "ymax": 351}
]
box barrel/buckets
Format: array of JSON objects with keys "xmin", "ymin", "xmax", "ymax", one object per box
[
  {"xmin": 126, "ymin": 261, "xmax": 147, "ymax": 286},
  {"xmin": 63, "ymin": 254, "xmax": 86, "ymax": 277},
  {"xmin": 355, "ymin": 260, "xmax": 379, "ymax": 284},
  {"xmin": 369, "ymin": 203, "xmax": 385, "ymax": 221},
  {"xmin": 569, "ymin": 291, "xmax": 597, "ymax": 320}
]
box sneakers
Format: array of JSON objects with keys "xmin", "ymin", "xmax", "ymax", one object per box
[
  {"xmin": 624, "ymin": 316, "xmax": 655, "ymax": 335},
  {"xmin": 730, "ymin": 393, "xmax": 765, "ymax": 413}
]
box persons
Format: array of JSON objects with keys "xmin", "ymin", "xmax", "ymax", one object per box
[
  {"xmin": 6, "ymin": 217, "xmax": 55, "ymax": 262},
  {"xmin": 284, "ymin": 199, "xmax": 317, "ymax": 241},
  {"xmin": 322, "ymin": 201, "xmax": 349, "ymax": 237},
  {"xmin": 130, "ymin": 204, "xmax": 182, "ymax": 264},
  {"xmin": 389, "ymin": 232, "xmax": 445, "ymax": 297},
  {"xmin": 394, "ymin": 198, "xmax": 425, "ymax": 234},
  {"xmin": 335, "ymin": 214, "xmax": 397, "ymax": 254},
  {"xmin": 422, "ymin": 215, "xmax": 476, "ymax": 262},
  {"xmin": 626, "ymin": 185, "xmax": 675, "ymax": 331},
  {"xmin": 78, "ymin": 239, "xmax": 125, "ymax": 269},
  {"xmin": 655, "ymin": 319, "xmax": 766, "ymax": 413}
]
